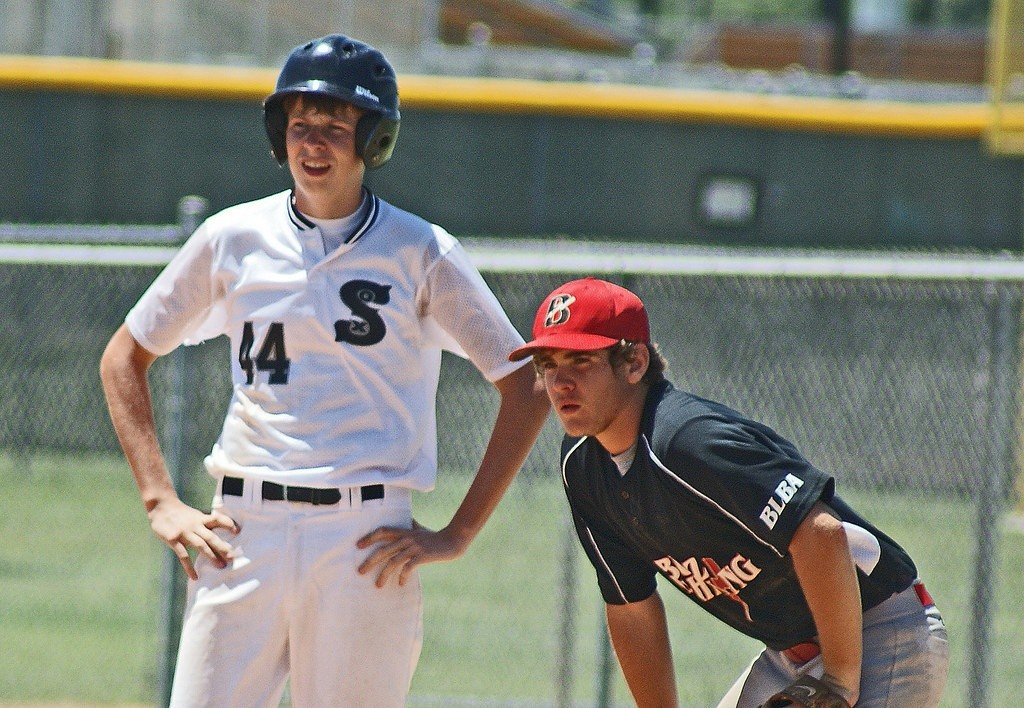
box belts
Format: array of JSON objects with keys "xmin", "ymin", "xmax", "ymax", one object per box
[
  {"xmin": 221, "ymin": 475, "xmax": 384, "ymax": 504},
  {"xmin": 784, "ymin": 580, "xmax": 935, "ymax": 668}
]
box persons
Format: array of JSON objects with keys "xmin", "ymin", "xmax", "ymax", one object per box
[
  {"xmin": 509, "ymin": 277, "xmax": 950, "ymax": 708},
  {"xmin": 98, "ymin": 34, "xmax": 551, "ymax": 708}
]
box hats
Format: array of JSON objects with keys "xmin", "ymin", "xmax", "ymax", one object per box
[{"xmin": 509, "ymin": 276, "xmax": 649, "ymax": 361}]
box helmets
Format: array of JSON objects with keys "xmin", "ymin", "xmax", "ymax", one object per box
[{"xmin": 262, "ymin": 34, "xmax": 401, "ymax": 169}]
